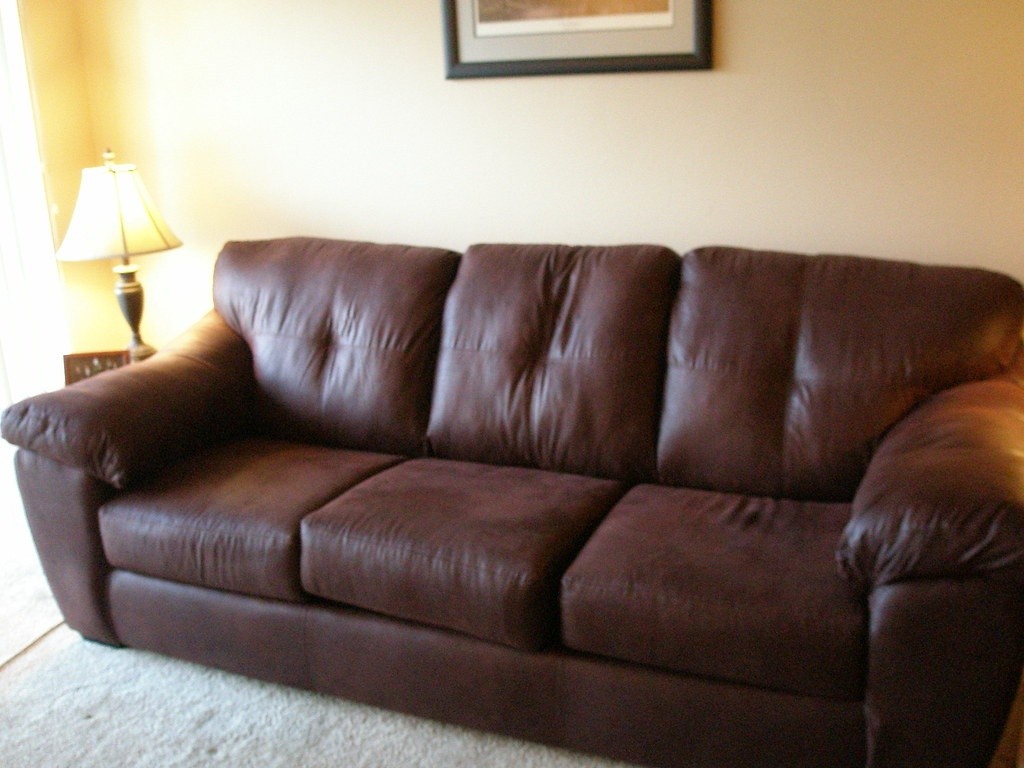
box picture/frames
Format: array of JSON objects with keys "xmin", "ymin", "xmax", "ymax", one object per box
[
  {"xmin": 63, "ymin": 349, "xmax": 131, "ymax": 385},
  {"xmin": 441, "ymin": 0, "xmax": 714, "ymax": 79}
]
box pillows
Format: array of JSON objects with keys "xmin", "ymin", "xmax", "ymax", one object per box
[
  {"xmin": 655, "ymin": 244, "xmax": 1024, "ymax": 502},
  {"xmin": 213, "ymin": 239, "xmax": 461, "ymax": 457},
  {"xmin": 423, "ymin": 243, "xmax": 682, "ymax": 482}
]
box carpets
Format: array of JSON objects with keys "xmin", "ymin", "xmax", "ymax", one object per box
[{"xmin": 0, "ymin": 639, "xmax": 627, "ymax": 768}]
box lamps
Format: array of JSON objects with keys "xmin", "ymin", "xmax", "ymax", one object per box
[{"xmin": 54, "ymin": 144, "xmax": 183, "ymax": 362}]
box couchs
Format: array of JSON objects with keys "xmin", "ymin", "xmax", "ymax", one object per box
[{"xmin": 0, "ymin": 235, "xmax": 1024, "ymax": 768}]
